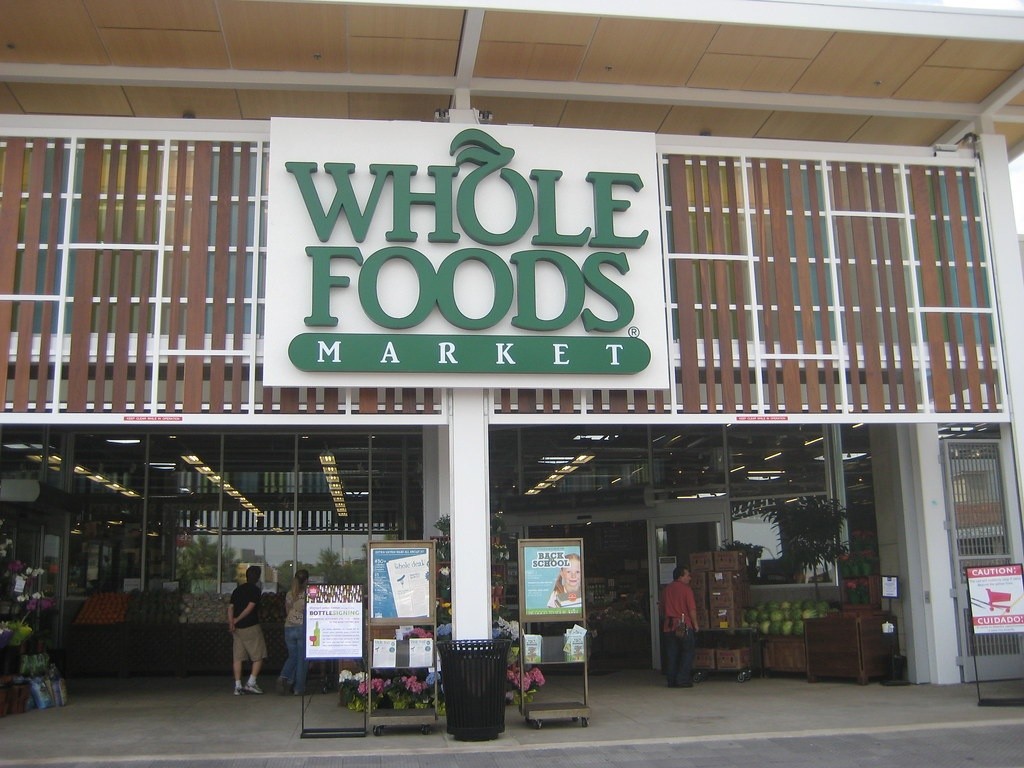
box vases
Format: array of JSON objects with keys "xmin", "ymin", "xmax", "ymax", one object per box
[
  {"xmin": 0, "ymin": 684, "xmax": 29, "ymax": 717},
  {"xmin": 439, "ymin": 583, "xmax": 451, "ymax": 601}
]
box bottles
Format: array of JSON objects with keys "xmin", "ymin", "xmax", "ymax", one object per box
[
  {"xmin": 588, "ymin": 583, "xmax": 606, "ymax": 604},
  {"xmin": 619, "ymin": 568, "xmax": 646, "ymax": 604},
  {"xmin": 607, "ymin": 577, "xmax": 617, "ymax": 602},
  {"xmin": 314, "ymin": 621, "xmax": 320, "ymax": 646}
]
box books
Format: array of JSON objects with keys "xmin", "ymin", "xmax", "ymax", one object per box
[{"xmin": 554, "ymin": 591, "xmax": 582, "ymax": 608}]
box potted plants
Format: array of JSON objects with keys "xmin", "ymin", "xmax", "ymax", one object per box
[
  {"xmin": 495, "ymin": 574, "xmax": 504, "ymax": 596},
  {"xmin": 32, "ymin": 630, "xmax": 55, "ymax": 653}
]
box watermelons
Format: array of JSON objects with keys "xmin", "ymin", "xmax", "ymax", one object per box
[{"xmin": 740, "ymin": 599, "xmax": 839, "ymax": 636}]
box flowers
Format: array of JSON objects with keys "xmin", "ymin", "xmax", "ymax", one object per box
[
  {"xmin": 6, "ymin": 559, "xmax": 45, "ymax": 580},
  {"xmin": 439, "ymin": 567, "xmax": 451, "ymax": 578},
  {"xmin": 0, "ymin": 538, "xmax": 13, "ymax": 558},
  {"xmin": 493, "ymin": 618, "xmax": 546, "ymax": 703},
  {"xmin": 17, "ymin": 591, "xmax": 54, "ymax": 610},
  {"xmin": 403, "ymin": 623, "xmax": 452, "ymax": 640},
  {"xmin": 339, "ymin": 670, "xmax": 445, "ymax": 701}
]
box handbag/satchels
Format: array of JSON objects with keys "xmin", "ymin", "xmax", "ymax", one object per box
[{"xmin": 675, "ymin": 621, "xmax": 688, "ymax": 644}]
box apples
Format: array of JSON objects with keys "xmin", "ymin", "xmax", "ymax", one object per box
[{"xmin": 73, "ymin": 590, "xmax": 130, "ymax": 624}]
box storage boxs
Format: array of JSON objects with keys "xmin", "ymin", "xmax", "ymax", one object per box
[
  {"xmin": 694, "ymin": 643, "xmax": 750, "ymax": 669},
  {"xmin": 687, "ymin": 550, "xmax": 744, "ymax": 630}
]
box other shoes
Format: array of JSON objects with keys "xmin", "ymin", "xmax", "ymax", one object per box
[
  {"xmin": 667, "ymin": 680, "xmax": 694, "ymax": 687},
  {"xmin": 277, "ymin": 676, "xmax": 296, "ymax": 688},
  {"xmin": 244, "ymin": 682, "xmax": 263, "ymax": 694},
  {"xmin": 234, "ymin": 686, "xmax": 245, "ymax": 695},
  {"xmin": 294, "ymin": 686, "xmax": 311, "ymax": 696},
  {"xmin": 319, "ymin": 676, "xmax": 325, "ymax": 682}
]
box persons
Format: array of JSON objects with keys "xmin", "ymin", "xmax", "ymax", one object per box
[
  {"xmin": 661, "ymin": 566, "xmax": 699, "ymax": 687},
  {"xmin": 277, "ymin": 570, "xmax": 309, "ymax": 696},
  {"xmin": 227, "ymin": 566, "xmax": 268, "ymax": 695},
  {"xmin": 547, "ymin": 553, "xmax": 580, "ymax": 609}
]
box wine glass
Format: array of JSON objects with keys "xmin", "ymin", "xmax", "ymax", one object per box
[{"xmin": 310, "ymin": 636, "xmax": 317, "ymax": 646}]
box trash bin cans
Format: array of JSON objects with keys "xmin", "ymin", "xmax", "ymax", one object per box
[
  {"xmin": 436, "ymin": 639, "xmax": 512, "ymax": 742},
  {"xmin": 877, "ymin": 656, "xmax": 909, "ymax": 686}
]
